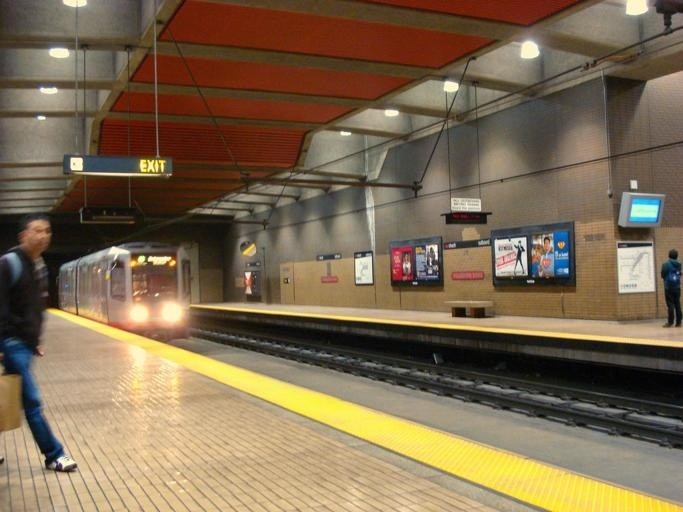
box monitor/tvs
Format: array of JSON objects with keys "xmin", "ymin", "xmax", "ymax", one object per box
[{"xmin": 619, "ymin": 193, "xmax": 666, "ymax": 228}]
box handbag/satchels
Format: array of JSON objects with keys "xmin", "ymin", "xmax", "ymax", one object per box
[{"xmin": 0, "ymin": 374, "xmax": 22, "ymax": 432}]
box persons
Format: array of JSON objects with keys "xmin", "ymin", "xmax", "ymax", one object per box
[
  {"xmin": 510, "ymin": 240, "xmax": 525, "ymax": 275},
  {"xmin": 0, "ymin": 211, "xmax": 79, "ymax": 475},
  {"xmin": 660, "ymin": 249, "xmax": 682, "ymax": 328},
  {"xmin": 539, "ymin": 237, "xmax": 554, "ymax": 277},
  {"xmin": 401, "ymin": 252, "xmax": 410, "ymax": 274}
]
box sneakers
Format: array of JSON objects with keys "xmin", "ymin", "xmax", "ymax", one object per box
[
  {"xmin": 661, "ymin": 323, "xmax": 672, "ymax": 328},
  {"xmin": 0, "ymin": 457, "xmax": 4, "ymax": 465},
  {"xmin": 675, "ymin": 324, "xmax": 680, "ymax": 327},
  {"xmin": 46, "ymin": 456, "xmax": 76, "ymax": 472}
]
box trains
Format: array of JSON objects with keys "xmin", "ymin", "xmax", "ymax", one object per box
[{"xmin": 57, "ymin": 237, "xmax": 195, "ymax": 341}]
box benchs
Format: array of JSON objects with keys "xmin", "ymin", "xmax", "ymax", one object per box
[{"xmin": 443, "ymin": 300, "xmax": 494, "ymax": 318}]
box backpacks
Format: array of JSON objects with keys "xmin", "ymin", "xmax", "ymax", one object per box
[{"xmin": 668, "ymin": 266, "xmax": 680, "ymax": 288}]
